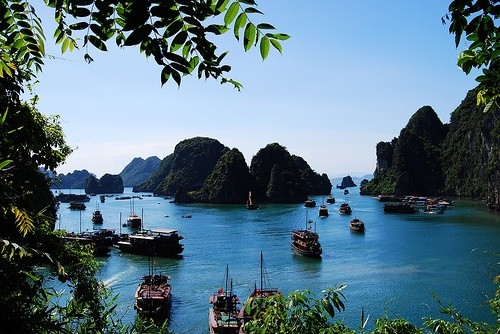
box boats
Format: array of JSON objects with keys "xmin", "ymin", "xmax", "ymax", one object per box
[
  {"xmin": 377, "ymin": 194, "xmax": 454, "ymax": 216},
  {"xmin": 349, "ymin": 216, "xmax": 366, "ymax": 232},
  {"xmin": 304, "ymin": 200, "xmax": 316, "ymax": 208},
  {"xmin": 245, "ymin": 202, "xmax": 262, "ymax": 210},
  {"xmin": 327, "ymin": 196, "xmax": 336, "ymax": 204},
  {"xmin": 343, "ymin": 189, "xmax": 348, "ymax": 195},
  {"xmin": 338, "ymin": 201, "xmax": 353, "ymax": 216}
]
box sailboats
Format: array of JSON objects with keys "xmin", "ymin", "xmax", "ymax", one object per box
[
  {"xmin": 58, "ymin": 190, "xmax": 185, "ymax": 256},
  {"xmin": 290, "ymin": 209, "xmax": 325, "ymax": 258},
  {"xmin": 127, "ymin": 198, "xmax": 142, "ymax": 229},
  {"xmin": 236, "ymin": 249, "xmax": 283, "ymax": 329},
  {"xmin": 131, "ymin": 256, "xmax": 172, "ymax": 321},
  {"xmin": 319, "ymin": 196, "xmax": 330, "ymax": 218},
  {"xmin": 207, "ymin": 264, "xmax": 241, "ymax": 334},
  {"xmin": 91, "ymin": 200, "xmax": 104, "ymax": 225}
]
